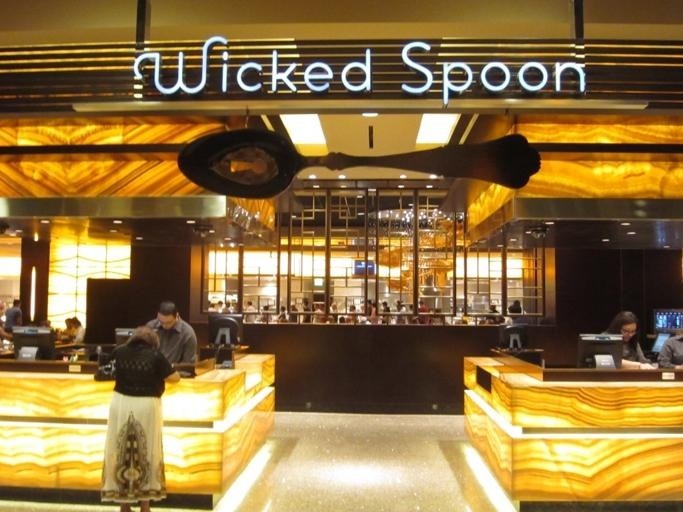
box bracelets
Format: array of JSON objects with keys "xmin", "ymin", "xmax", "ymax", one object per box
[{"xmin": 637, "ymin": 361, "xmax": 642, "ymax": 369}]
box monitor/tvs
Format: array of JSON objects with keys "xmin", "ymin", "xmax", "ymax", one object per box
[
  {"xmin": 115, "ymin": 328, "xmax": 160, "ymax": 346},
  {"xmin": 649, "ymin": 332, "xmax": 676, "ymax": 355},
  {"xmin": 576, "ymin": 334, "xmax": 624, "ymax": 369},
  {"xmin": 12, "ymin": 326, "xmax": 56, "ymax": 360},
  {"xmin": 209, "ymin": 314, "xmax": 244, "ymax": 348},
  {"xmin": 497, "ymin": 323, "xmax": 528, "ymax": 349},
  {"xmin": 653, "ymin": 309, "xmax": 683, "ymax": 330},
  {"xmin": 352, "ymin": 259, "xmax": 376, "ymax": 275}
]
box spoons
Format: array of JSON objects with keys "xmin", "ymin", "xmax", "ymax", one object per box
[{"xmin": 176, "ymin": 127, "xmax": 543, "ymax": 200}]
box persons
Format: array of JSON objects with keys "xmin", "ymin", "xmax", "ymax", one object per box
[
  {"xmin": 95, "ymin": 326, "xmax": 179, "ymax": 511},
  {"xmin": 0, "ymin": 298, "xmax": 86, "ymax": 349},
  {"xmin": 600, "ymin": 310, "xmax": 655, "ymax": 370},
  {"xmin": 143, "ymin": 302, "xmax": 197, "ymax": 367},
  {"xmin": 207, "ymin": 298, "xmax": 520, "ymax": 324},
  {"xmin": 657, "ymin": 334, "xmax": 682, "ymax": 370}
]
